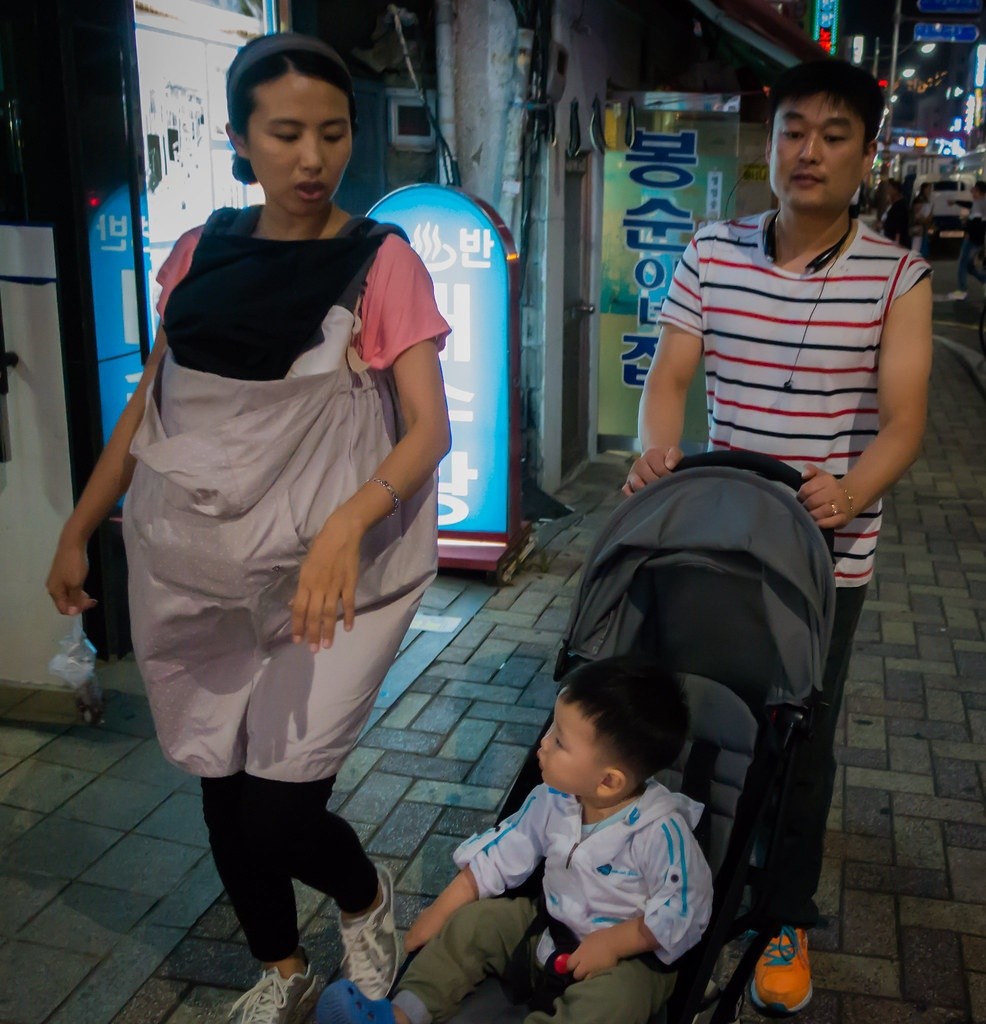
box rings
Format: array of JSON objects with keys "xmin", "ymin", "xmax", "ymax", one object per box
[{"xmin": 831, "ymin": 503, "xmax": 838, "ymax": 514}]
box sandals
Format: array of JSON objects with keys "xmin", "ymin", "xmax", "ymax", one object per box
[{"xmin": 315, "ymin": 978, "xmax": 396, "ymax": 1024}]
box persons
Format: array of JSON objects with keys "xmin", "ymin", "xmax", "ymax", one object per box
[
  {"xmin": 44, "ymin": 34, "xmax": 986, "ymax": 1024},
  {"xmin": 316, "ymin": 655, "xmax": 714, "ymax": 1024}
]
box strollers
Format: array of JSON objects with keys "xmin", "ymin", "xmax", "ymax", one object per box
[{"xmin": 382, "ymin": 449, "xmax": 839, "ymax": 1024}]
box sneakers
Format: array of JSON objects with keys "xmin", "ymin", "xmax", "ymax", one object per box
[
  {"xmin": 748, "ymin": 925, "xmax": 813, "ymax": 1012},
  {"xmin": 338, "ymin": 864, "xmax": 399, "ymax": 1000},
  {"xmin": 228, "ymin": 947, "xmax": 318, "ymax": 1024}
]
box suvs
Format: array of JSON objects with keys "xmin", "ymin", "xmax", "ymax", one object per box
[{"xmin": 896, "ymin": 173, "xmax": 985, "ymax": 241}]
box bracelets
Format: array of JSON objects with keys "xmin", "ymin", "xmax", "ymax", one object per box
[
  {"xmin": 370, "ymin": 477, "xmax": 399, "ymax": 515},
  {"xmin": 844, "ymin": 484, "xmax": 852, "ymax": 517}
]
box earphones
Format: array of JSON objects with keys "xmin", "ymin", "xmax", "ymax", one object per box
[{"xmin": 783, "ymin": 380, "xmax": 793, "ymax": 390}]
